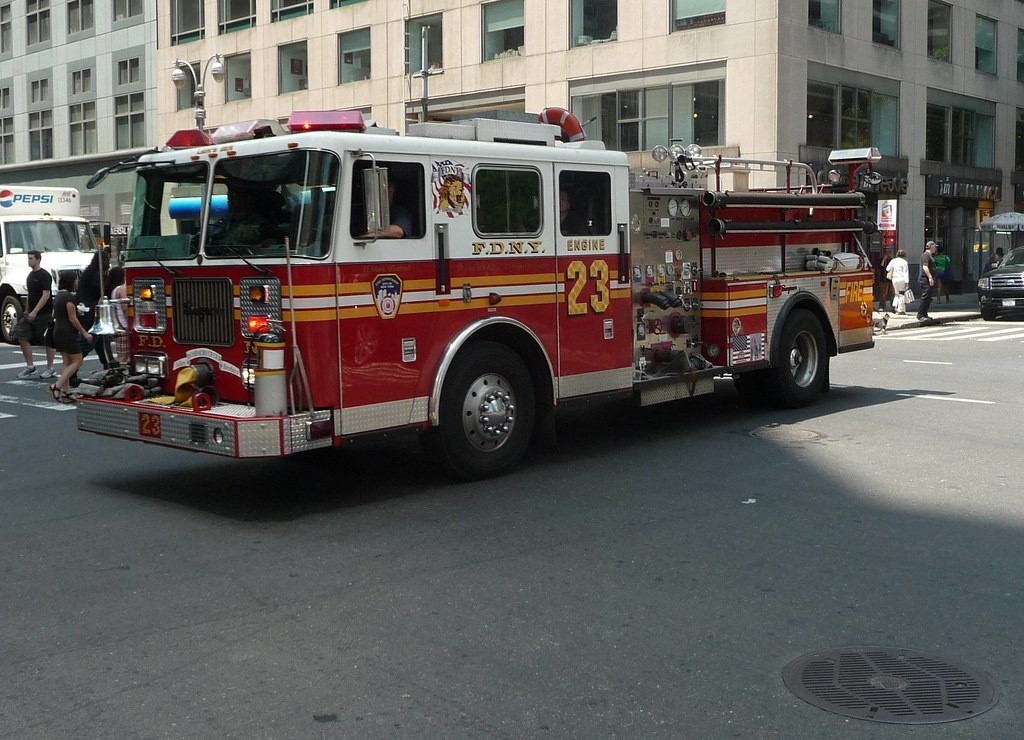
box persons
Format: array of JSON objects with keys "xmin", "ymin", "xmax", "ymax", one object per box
[
  {"xmin": 558, "ymin": 186, "xmax": 586, "ymax": 237},
  {"xmin": 15, "ymin": 250, "xmax": 56, "ymax": 379},
  {"xmin": 108, "ymin": 264, "xmax": 130, "ymax": 363},
  {"xmin": 990, "ymin": 247, "xmax": 1005, "ymax": 265},
  {"xmin": 934, "ymin": 247, "xmax": 953, "ymax": 304},
  {"xmin": 916, "ymin": 241, "xmax": 939, "ymax": 322},
  {"xmin": 70, "ymin": 250, "xmax": 111, "ymax": 387},
  {"xmin": 49, "ymin": 270, "xmax": 93, "ymax": 404},
  {"xmin": 886, "ymin": 249, "xmax": 909, "ymax": 315},
  {"xmin": 877, "ymin": 249, "xmax": 897, "ymax": 315},
  {"xmin": 359, "ymin": 181, "xmax": 413, "ymax": 237}
]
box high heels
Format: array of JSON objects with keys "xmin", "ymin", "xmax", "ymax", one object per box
[
  {"xmin": 61, "ymin": 392, "xmax": 77, "ymax": 404},
  {"xmin": 46, "ymin": 384, "xmax": 62, "ymax": 402}
]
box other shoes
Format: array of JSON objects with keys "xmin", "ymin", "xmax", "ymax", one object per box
[
  {"xmin": 947, "ymin": 300, "xmax": 953, "ymax": 304},
  {"xmin": 898, "ymin": 312, "xmax": 906, "ymax": 315},
  {"xmin": 918, "ymin": 317, "xmax": 932, "ymax": 322},
  {"xmin": 937, "ymin": 300, "xmax": 943, "ymax": 304},
  {"xmin": 892, "ymin": 306, "xmax": 896, "ymax": 314}
]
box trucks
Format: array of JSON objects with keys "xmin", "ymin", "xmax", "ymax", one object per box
[{"xmin": 1, "ymin": 185, "xmax": 111, "ymax": 345}]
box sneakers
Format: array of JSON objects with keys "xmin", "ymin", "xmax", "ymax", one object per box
[
  {"xmin": 17, "ymin": 366, "xmax": 39, "ymax": 378},
  {"xmin": 40, "ymin": 368, "xmax": 57, "ymax": 378}
]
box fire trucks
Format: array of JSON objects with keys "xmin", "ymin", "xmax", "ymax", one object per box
[{"xmin": 68, "ymin": 108, "xmax": 891, "ymax": 482}]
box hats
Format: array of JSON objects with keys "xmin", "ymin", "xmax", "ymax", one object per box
[{"xmin": 926, "ymin": 241, "xmax": 938, "ymax": 249}]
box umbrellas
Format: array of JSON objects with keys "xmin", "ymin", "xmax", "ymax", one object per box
[{"xmin": 979, "ymin": 211, "xmax": 1024, "ymax": 249}]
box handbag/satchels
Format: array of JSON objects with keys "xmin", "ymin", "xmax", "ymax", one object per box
[
  {"xmin": 904, "ymin": 288, "xmax": 914, "ymax": 303},
  {"xmin": 887, "ymin": 259, "xmax": 894, "ymax": 280},
  {"xmin": 941, "ymin": 270, "xmax": 954, "ymax": 282}
]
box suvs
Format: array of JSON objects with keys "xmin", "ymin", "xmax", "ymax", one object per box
[{"xmin": 977, "ymin": 246, "xmax": 1024, "ymax": 321}]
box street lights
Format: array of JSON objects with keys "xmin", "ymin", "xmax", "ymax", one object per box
[{"xmin": 171, "ymin": 52, "xmax": 228, "ymax": 229}]
